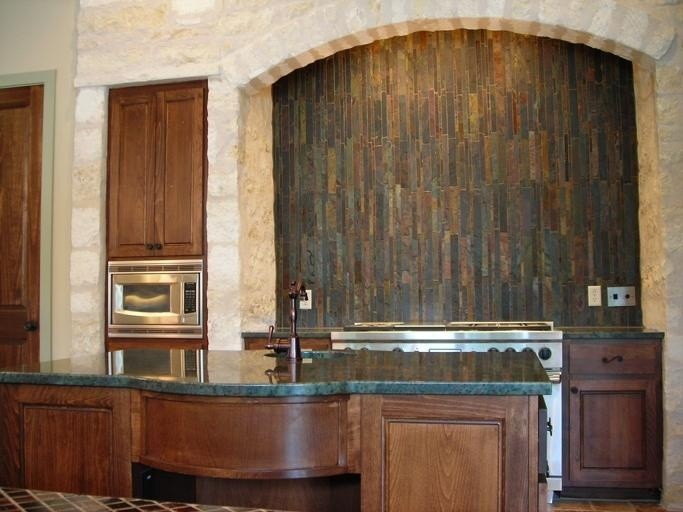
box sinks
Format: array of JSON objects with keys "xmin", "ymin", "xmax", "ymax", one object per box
[{"xmin": 263, "ymin": 350, "xmax": 357, "ymax": 361}]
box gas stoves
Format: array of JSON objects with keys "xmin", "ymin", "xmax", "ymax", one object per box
[{"xmin": 343, "ymin": 322, "xmax": 553, "ymax": 331}]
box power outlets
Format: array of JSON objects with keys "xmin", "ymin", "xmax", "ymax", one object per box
[
  {"xmin": 587, "ymin": 286, "xmax": 601, "ymax": 307},
  {"xmin": 299, "ymin": 289, "xmax": 311, "ymax": 310}
]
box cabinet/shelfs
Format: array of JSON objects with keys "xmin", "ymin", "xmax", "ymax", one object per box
[
  {"xmin": 104, "ymin": 79, "xmax": 206, "ymax": 260},
  {"xmin": 0, "ymin": 83, "xmax": 41, "ymax": 365},
  {"xmin": 562, "ymin": 339, "xmax": 662, "ymax": 501}
]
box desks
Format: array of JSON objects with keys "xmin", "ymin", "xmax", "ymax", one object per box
[{"xmin": 0, "ymin": 351, "xmax": 552, "ymax": 512}]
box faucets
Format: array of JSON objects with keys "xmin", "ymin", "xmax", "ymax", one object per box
[
  {"xmin": 265, "ymin": 281, "xmax": 309, "ymax": 359},
  {"xmin": 265, "ymin": 358, "xmax": 303, "ymax": 385}
]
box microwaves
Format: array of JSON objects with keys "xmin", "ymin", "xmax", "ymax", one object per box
[
  {"xmin": 107, "ymin": 260, "xmax": 203, "ymax": 340},
  {"xmin": 106, "ymin": 348, "xmax": 204, "ymax": 387}
]
